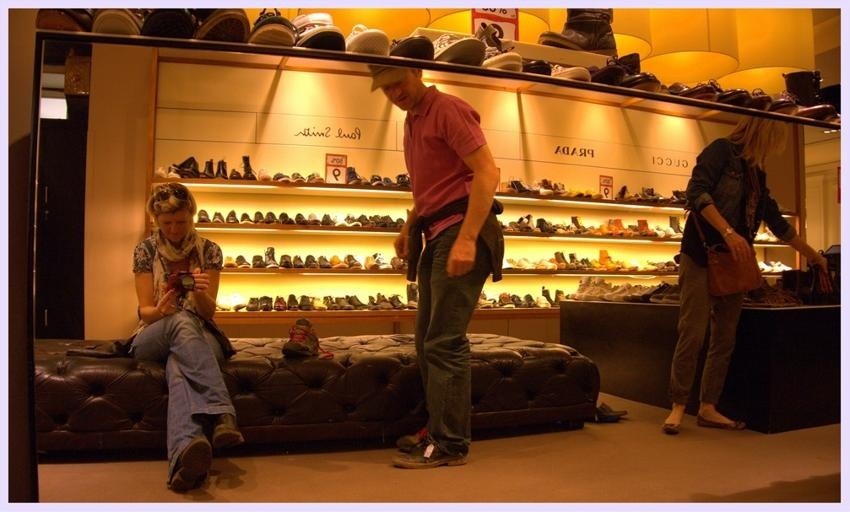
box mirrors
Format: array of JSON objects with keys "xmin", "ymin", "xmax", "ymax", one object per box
[{"xmin": 28, "ymin": 29, "xmax": 841, "ymax": 503}]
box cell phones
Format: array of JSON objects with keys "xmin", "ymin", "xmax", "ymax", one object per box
[{"xmin": 176, "ymin": 270, "xmax": 195, "ymax": 298}]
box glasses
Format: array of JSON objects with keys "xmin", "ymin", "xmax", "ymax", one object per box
[{"xmin": 160, "ymin": 189, "xmax": 187, "ymax": 200}]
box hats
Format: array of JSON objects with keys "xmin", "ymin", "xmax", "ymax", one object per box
[{"xmin": 369, "ymin": 64, "xmax": 406, "ymax": 91}]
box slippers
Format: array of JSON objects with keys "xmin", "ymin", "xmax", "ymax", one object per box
[{"xmin": 594, "ymin": 403, "xmax": 627, "ymax": 424}]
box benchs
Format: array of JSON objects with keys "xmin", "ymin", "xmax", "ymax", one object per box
[{"xmin": 33, "ymin": 334, "xmax": 600, "ymax": 452}]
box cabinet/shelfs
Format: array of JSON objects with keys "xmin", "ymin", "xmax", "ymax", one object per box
[{"xmin": 149, "ymin": 175, "xmax": 802, "ymax": 344}]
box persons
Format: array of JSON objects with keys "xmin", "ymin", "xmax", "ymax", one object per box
[
  {"xmin": 129, "ymin": 184, "xmax": 245, "ymax": 493},
  {"xmin": 659, "ymin": 115, "xmax": 834, "ymax": 433},
  {"xmin": 361, "ymin": 63, "xmax": 505, "ymax": 469}
]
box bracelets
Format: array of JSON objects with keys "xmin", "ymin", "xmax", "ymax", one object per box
[{"xmin": 724, "ymin": 228, "xmax": 734, "ymax": 240}]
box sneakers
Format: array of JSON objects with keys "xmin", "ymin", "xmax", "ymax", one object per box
[
  {"xmin": 757, "ymin": 261, "xmax": 793, "ymax": 273},
  {"xmin": 477, "ymin": 178, "xmax": 687, "ymax": 308},
  {"xmin": 282, "ymin": 319, "xmax": 319, "ymax": 357}
]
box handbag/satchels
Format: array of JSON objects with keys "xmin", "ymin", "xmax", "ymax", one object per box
[{"xmin": 708, "ymin": 243, "xmax": 764, "ymax": 298}]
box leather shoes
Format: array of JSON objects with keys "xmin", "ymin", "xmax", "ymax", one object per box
[
  {"xmin": 391, "ymin": 428, "xmax": 470, "ymax": 469},
  {"xmin": 664, "ymin": 422, "xmax": 680, "ymax": 434},
  {"xmin": 697, "ymin": 413, "xmax": 746, "ymax": 431},
  {"xmin": 199, "ymin": 157, "xmax": 419, "ymax": 311},
  {"xmin": 171, "ymin": 414, "xmax": 245, "ymax": 492}
]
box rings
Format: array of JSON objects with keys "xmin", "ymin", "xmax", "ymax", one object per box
[{"xmin": 170, "ymin": 303, "xmax": 177, "ymax": 310}]
